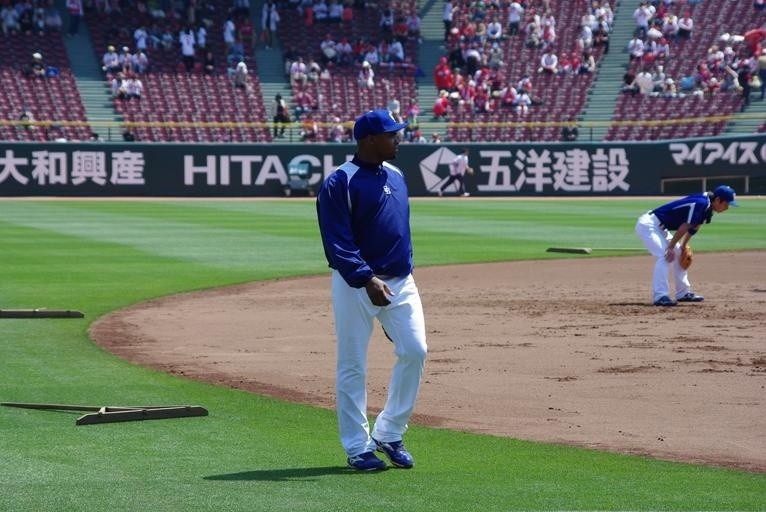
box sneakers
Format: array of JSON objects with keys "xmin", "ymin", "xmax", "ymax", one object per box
[
  {"xmin": 654, "ymin": 296, "xmax": 677, "ymax": 306},
  {"xmin": 677, "ymin": 293, "xmax": 704, "ymax": 301},
  {"xmin": 347, "ymin": 452, "xmax": 387, "ymax": 471},
  {"xmin": 372, "ymin": 437, "xmax": 415, "ymax": 468}
]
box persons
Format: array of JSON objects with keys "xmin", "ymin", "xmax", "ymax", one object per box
[
  {"xmin": 58, "ymin": 131, "xmax": 135, "ymax": 143},
  {"xmin": 260, "ymin": 1, "xmax": 440, "ymax": 144},
  {"xmin": 1, "ymin": 1, "xmax": 85, "ymax": 130},
  {"xmin": 316, "ymin": 108, "xmax": 428, "ymax": 471},
  {"xmin": 635, "ymin": 185, "xmax": 740, "ymax": 306},
  {"xmin": 90, "ymin": 1, "xmax": 252, "ymax": 101},
  {"xmin": 432, "ymin": 1, "xmax": 619, "ymax": 144},
  {"xmin": 622, "ymin": 0, "xmax": 764, "ymax": 106}
]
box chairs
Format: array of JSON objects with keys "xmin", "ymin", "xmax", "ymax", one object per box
[{"xmin": 1, "ymin": 1, "xmax": 765, "ymax": 144}]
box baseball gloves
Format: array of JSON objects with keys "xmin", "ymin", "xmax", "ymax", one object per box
[{"xmin": 680, "ymin": 246, "xmax": 693, "ymax": 270}]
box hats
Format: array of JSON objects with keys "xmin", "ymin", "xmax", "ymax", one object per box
[
  {"xmin": 713, "ymin": 185, "xmax": 740, "ymax": 207},
  {"xmin": 354, "ymin": 110, "xmax": 409, "ymax": 140}
]
